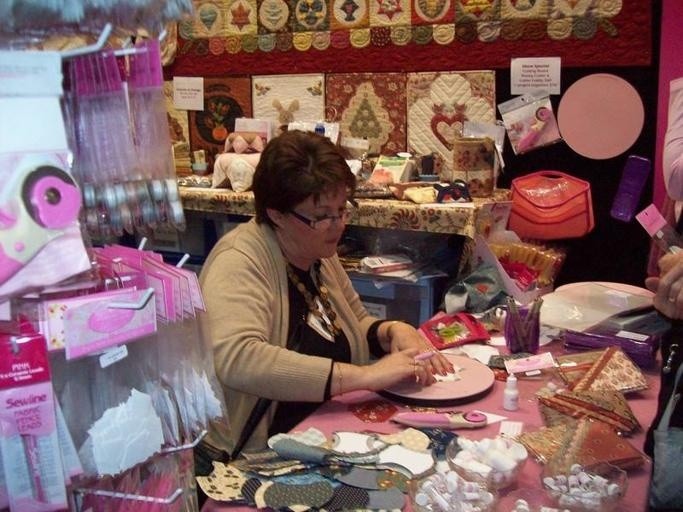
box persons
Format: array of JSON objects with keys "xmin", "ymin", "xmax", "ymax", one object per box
[
  {"xmin": 661, "ymin": 76, "xmax": 682, "ymax": 235},
  {"xmin": 644, "ymin": 248, "xmax": 682, "ymax": 457},
  {"xmin": 194, "ymin": 128, "xmax": 455, "ymax": 508}
]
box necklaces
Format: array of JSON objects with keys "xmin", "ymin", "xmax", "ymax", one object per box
[{"xmin": 281, "ymin": 249, "xmax": 344, "ymax": 337}]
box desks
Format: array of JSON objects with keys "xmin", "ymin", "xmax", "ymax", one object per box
[
  {"xmin": 179, "ymin": 173, "xmax": 513, "ymax": 282},
  {"xmin": 201, "ymin": 314, "xmax": 664, "ymax": 508}
]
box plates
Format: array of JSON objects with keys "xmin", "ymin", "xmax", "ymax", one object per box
[
  {"xmin": 554, "ymin": 280, "xmax": 655, "ymax": 315},
  {"xmin": 378, "ymin": 350, "xmax": 496, "ymax": 401}
]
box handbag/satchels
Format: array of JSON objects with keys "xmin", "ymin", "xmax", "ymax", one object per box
[{"xmin": 506, "ymin": 169, "xmax": 595, "ymax": 242}]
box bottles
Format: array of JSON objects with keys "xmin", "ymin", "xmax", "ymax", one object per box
[
  {"xmin": 408, "ymin": 432, "xmax": 531, "ymax": 511},
  {"xmin": 539, "ymin": 463, "xmax": 618, "ymax": 512},
  {"xmin": 502, "ymin": 372, "xmax": 520, "ymax": 412}
]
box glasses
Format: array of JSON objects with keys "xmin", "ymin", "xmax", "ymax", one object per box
[{"xmin": 284, "ymin": 194, "xmax": 359, "ymax": 231}]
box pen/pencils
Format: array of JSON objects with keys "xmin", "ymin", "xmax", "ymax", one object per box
[
  {"xmin": 506, "ymin": 294, "xmax": 544, "ymax": 352},
  {"xmin": 414, "ymin": 351, "xmax": 436, "ymax": 360}
]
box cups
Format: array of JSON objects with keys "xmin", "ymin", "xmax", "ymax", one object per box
[{"xmin": 504, "ymin": 304, "xmax": 540, "ymax": 354}]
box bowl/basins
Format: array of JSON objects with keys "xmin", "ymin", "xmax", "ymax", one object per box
[{"xmin": 540, "ymin": 456, "xmax": 629, "ymax": 512}]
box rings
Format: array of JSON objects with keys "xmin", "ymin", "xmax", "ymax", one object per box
[
  {"xmin": 668, "ymin": 297, "xmax": 677, "ymax": 304},
  {"xmin": 414, "ymin": 365, "xmax": 418, "ymax": 376},
  {"xmin": 414, "ymin": 358, "xmax": 420, "ymax": 367}
]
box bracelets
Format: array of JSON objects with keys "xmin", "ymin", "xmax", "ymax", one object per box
[{"xmin": 336, "ymin": 360, "xmax": 344, "ymax": 399}]
